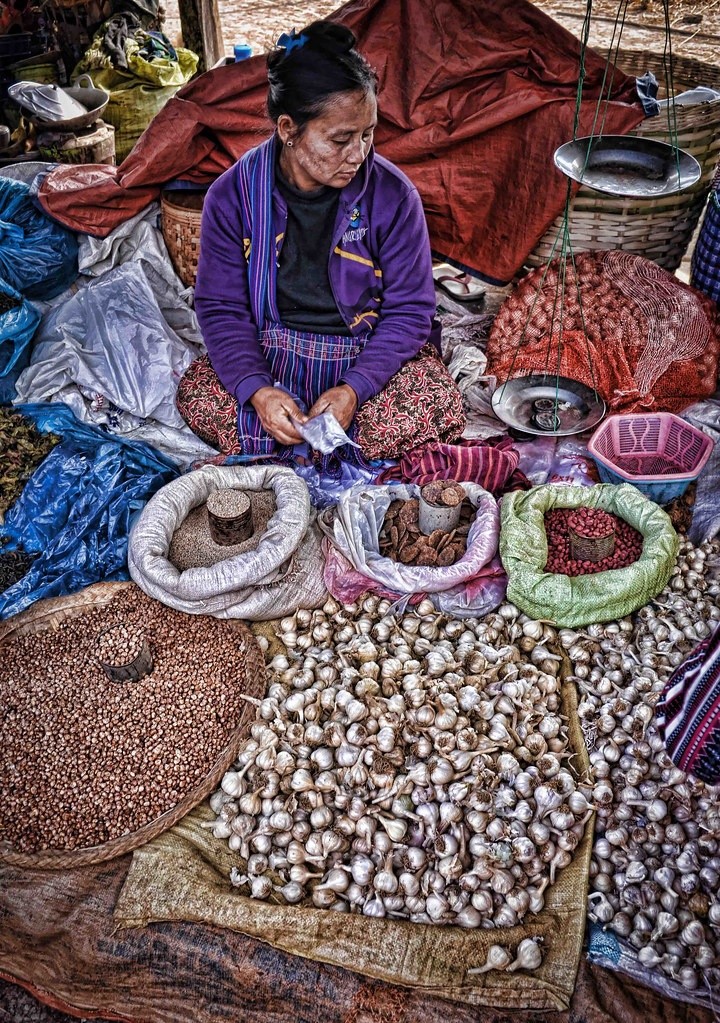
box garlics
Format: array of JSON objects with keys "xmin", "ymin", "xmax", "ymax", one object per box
[
  {"xmin": 199, "ymin": 534, "xmax": 720, "ymax": 998},
  {"xmin": 486, "ymin": 249, "xmax": 720, "ymax": 404}
]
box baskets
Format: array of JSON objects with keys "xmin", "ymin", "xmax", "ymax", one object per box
[{"xmin": 587, "ymin": 412, "xmax": 714, "ymax": 503}]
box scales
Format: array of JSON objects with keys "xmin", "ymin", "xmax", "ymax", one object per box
[{"xmin": 486, "ymin": 0, "xmax": 707, "ymax": 438}]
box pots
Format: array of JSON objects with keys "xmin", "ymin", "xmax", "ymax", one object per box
[{"xmin": 19, "ymin": 73, "xmax": 109, "ymax": 132}]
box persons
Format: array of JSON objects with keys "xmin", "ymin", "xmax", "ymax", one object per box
[{"xmin": 176, "ymin": 21, "xmax": 466, "ymax": 471}]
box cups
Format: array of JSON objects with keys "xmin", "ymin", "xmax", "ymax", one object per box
[
  {"xmin": 207, "ymin": 488, "xmax": 254, "ymax": 546},
  {"xmin": 567, "ymin": 521, "xmax": 615, "ymax": 562},
  {"xmin": 420, "ymin": 480, "xmax": 464, "ymax": 537},
  {"xmin": 97, "ymin": 623, "xmax": 154, "ymax": 683}
]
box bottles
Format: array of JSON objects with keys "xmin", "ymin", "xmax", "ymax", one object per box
[{"xmin": 234, "ymin": 45, "xmax": 252, "ymax": 64}]
